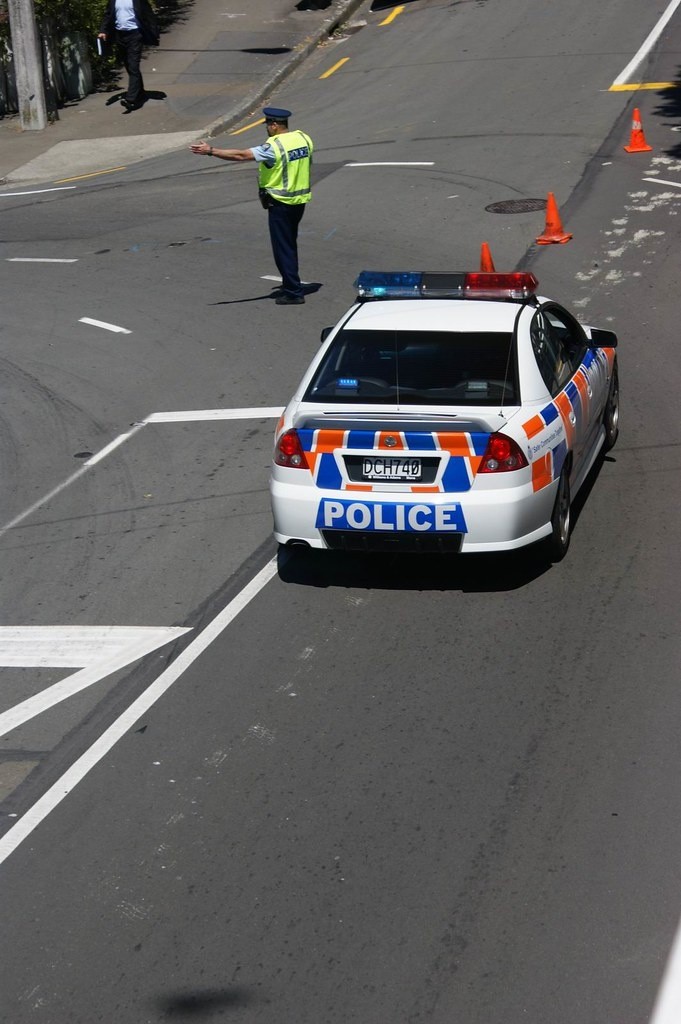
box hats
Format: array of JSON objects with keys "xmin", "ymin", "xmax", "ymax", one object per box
[{"xmin": 262, "ymin": 108, "xmax": 291, "ymax": 124}]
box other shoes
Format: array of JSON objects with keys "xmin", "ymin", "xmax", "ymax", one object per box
[
  {"xmin": 142, "ymin": 92, "xmax": 149, "ymax": 102},
  {"xmin": 121, "ymin": 98, "xmax": 137, "ymax": 110},
  {"xmin": 271, "ymin": 290, "xmax": 284, "ymax": 298},
  {"xmin": 275, "ymin": 296, "xmax": 305, "ymax": 304}
]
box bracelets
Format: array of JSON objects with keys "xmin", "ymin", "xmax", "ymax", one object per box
[{"xmin": 208, "ymin": 146, "xmax": 213, "ymax": 157}]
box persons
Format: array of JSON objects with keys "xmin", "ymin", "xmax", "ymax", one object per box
[
  {"xmin": 98, "ymin": 0, "xmax": 160, "ymax": 110},
  {"xmin": 189, "ymin": 107, "xmax": 314, "ymax": 305}
]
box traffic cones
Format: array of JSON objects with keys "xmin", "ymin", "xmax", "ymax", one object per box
[
  {"xmin": 623, "ymin": 108, "xmax": 653, "ymax": 152},
  {"xmin": 479, "ymin": 243, "xmax": 497, "ymax": 275},
  {"xmin": 534, "ymin": 192, "xmax": 575, "ymax": 244}
]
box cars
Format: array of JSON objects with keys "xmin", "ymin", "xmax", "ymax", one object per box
[{"xmin": 269, "ymin": 270, "xmax": 622, "ymax": 579}]
